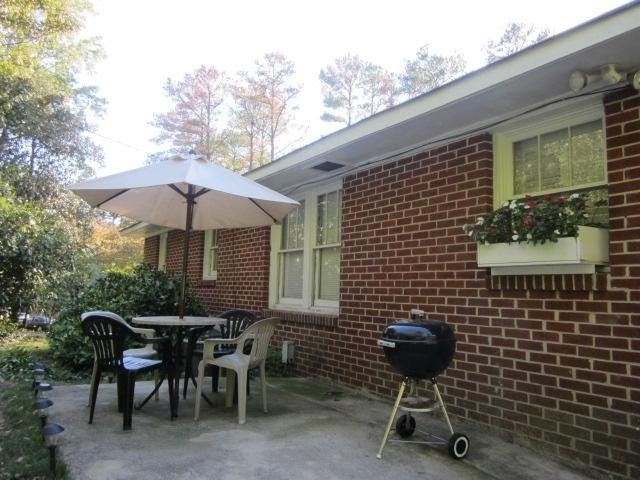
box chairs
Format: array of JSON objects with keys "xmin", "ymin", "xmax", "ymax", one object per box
[
  {"xmin": 194, "ymin": 316, "xmax": 280, "ymax": 424},
  {"xmin": 82, "ymin": 311, "xmax": 160, "ymax": 407},
  {"xmin": 79, "ymin": 317, "xmax": 178, "ymax": 431},
  {"xmin": 183, "ymin": 311, "xmax": 260, "ymax": 400}
]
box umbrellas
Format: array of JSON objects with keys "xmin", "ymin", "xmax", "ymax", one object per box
[{"xmin": 67, "ymin": 148, "xmax": 302, "ymax": 417}]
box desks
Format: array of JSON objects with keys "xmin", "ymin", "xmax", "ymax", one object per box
[{"xmin": 133, "ymin": 317, "xmax": 227, "ymax": 416}]
